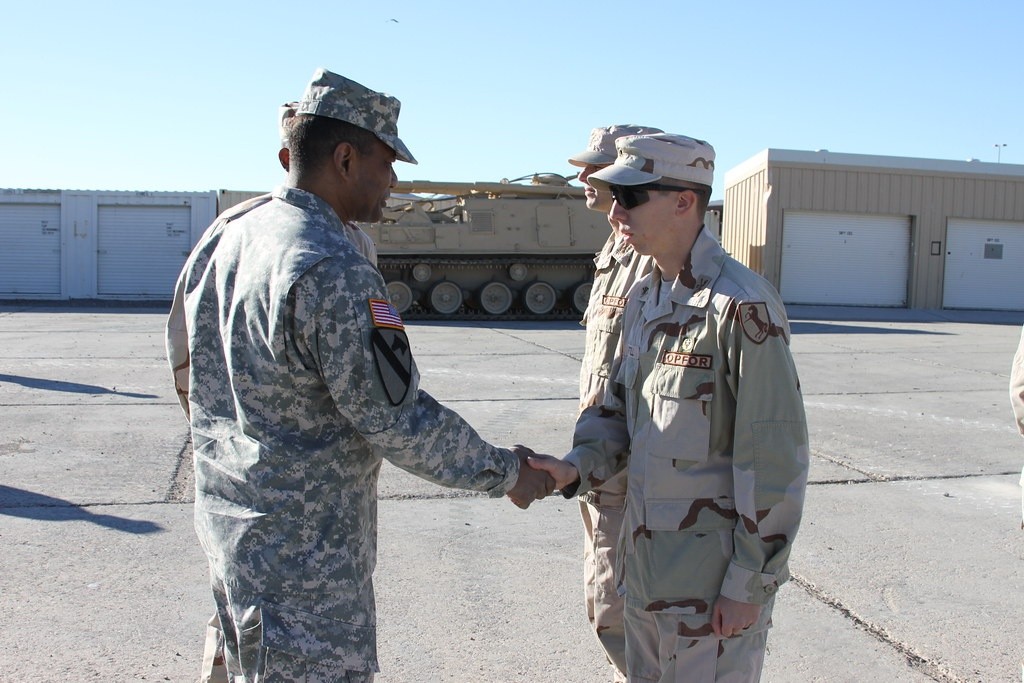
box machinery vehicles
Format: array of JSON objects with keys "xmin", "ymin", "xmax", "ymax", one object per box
[{"xmin": 354, "ymin": 171, "xmax": 611, "ymax": 321}]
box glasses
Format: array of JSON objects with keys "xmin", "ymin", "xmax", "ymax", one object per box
[{"xmin": 608, "ymin": 183, "xmax": 706, "ymax": 210}]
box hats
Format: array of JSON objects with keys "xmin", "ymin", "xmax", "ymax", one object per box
[
  {"xmin": 278, "ymin": 100, "xmax": 301, "ymax": 141},
  {"xmin": 568, "ymin": 124, "xmax": 666, "ymax": 167},
  {"xmin": 587, "ymin": 133, "xmax": 716, "ymax": 192},
  {"xmin": 297, "ymin": 67, "xmax": 419, "ymax": 166}
]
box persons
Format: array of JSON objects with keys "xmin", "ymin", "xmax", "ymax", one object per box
[
  {"xmin": 525, "ymin": 133, "xmax": 811, "ymax": 683},
  {"xmin": 162, "ymin": 71, "xmax": 559, "ymax": 683},
  {"xmin": 567, "ymin": 123, "xmax": 667, "ymax": 679}
]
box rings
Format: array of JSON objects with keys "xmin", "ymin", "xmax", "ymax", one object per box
[{"xmin": 743, "ymin": 624, "xmax": 751, "ymax": 630}]
